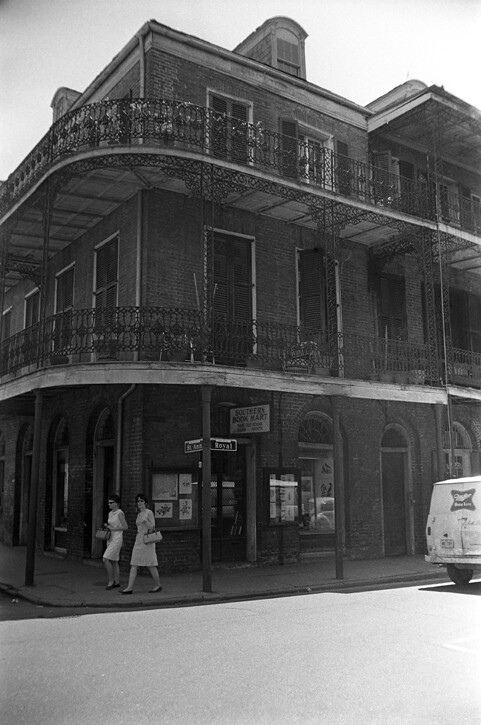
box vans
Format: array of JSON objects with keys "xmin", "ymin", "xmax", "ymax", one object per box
[
  {"xmin": 211, "ymin": 480, "xmax": 299, "ymax": 538},
  {"xmin": 424, "ymin": 475, "xmax": 481, "ymax": 585}
]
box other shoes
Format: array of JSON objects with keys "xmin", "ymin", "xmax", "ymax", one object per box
[
  {"xmin": 118, "ymin": 589, "xmax": 132, "ymax": 594},
  {"xmin": 148, "ymin": 586, "xmax": 162, "ymax": 594},
  {"xmin": 105, "ymin": 580, "xmax": 115, "ymax": 590},
  {"xmin": 115, "ymin": 584, "xmax": 120, "ymax": 588}
]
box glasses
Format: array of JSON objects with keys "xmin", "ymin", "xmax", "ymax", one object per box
[
  {"xmin": 107, "ymin": 502, "xmax": 114, "ymax": 504},
  {"xmin": 137, "ymin": 500, "xmax": 144, "ymax": 502}
]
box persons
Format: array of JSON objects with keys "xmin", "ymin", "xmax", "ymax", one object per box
[
  {"xmin": 119, "ymin": 493, "xmax": 163, "ymax": 594},
  {"xmin": 95, "ymin": 494, "xmax": 128, "ymax": 590}
]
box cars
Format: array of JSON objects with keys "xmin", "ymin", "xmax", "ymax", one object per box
[{"xmin": 307, "ymin": 497, "xmax": 335, "ymax": 531}]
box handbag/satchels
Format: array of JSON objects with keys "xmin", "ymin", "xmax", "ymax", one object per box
[
  {"xmin": 96, "ymin": 523, "xmax": 111, "ymax": 540},
  {"xmin": 143, "ymin": 525, "xmax": 163, "ymax": 544}
]
